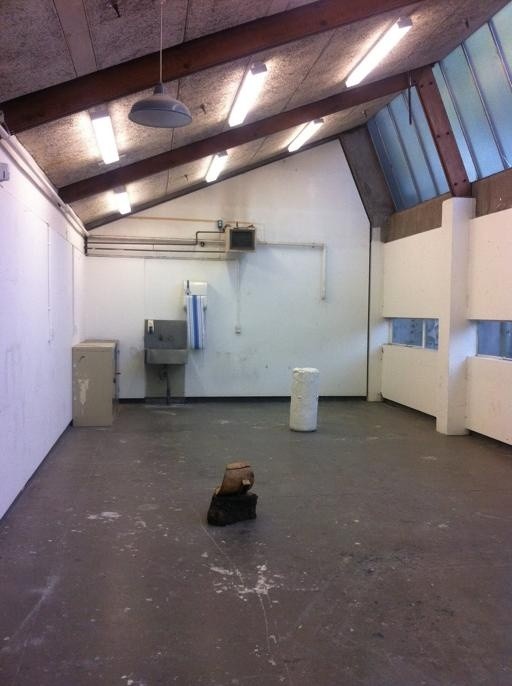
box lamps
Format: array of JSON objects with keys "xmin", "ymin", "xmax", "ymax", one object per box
[
  {"xmin": 89, "ymin": 111, "xmax": 119, "ymax": 164},
  {"xmin": 205, "ymin": 151, "xmax": 228, "ymax": 183},
  {"xmin": 228, "ymin": 62, "xmax": 267, "ymax": 127},
  {"xmin": 345, "ymin": 16, "xmax": 412, "ymax": 87},
  {"xmin": 113, "ymin": 184, "xmax": 131, "ymax": 216},
  {"xmin": 128, "ymin": 1, "xmax": 193, "ymax": 129},
  {"xmin": 288, "ymin": 118, "xmax": 324, "ymax": 152}
]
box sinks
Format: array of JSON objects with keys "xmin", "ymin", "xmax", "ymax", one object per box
[{"xmin": 146, "ymin": 349, "xmax": 187, "ymax": 365}]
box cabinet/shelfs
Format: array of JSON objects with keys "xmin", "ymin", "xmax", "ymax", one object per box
[{"xmin": 72, "ymin": 339, "xmax": 119, "ymax": 427}]
